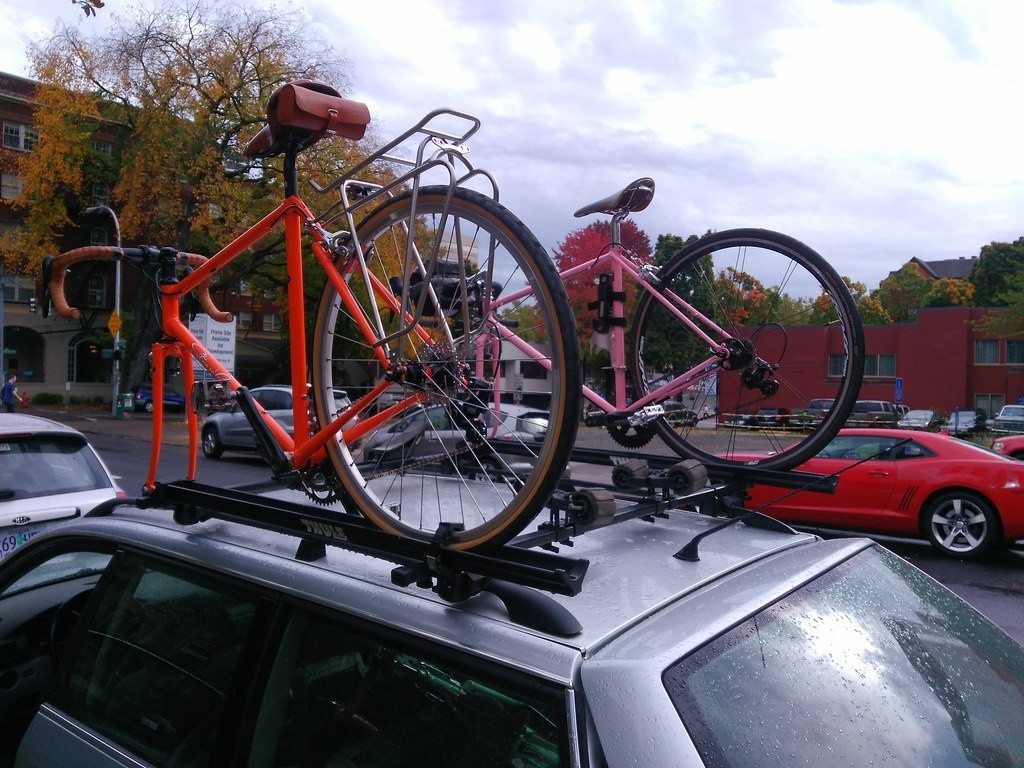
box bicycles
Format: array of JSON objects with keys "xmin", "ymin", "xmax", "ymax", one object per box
[
  {"xmin": 402, "ymin": 175, "xmax": 869, "ymax": 469},
  {"xmin": 32, "ymin": 79, "xmax": 587, "ymax": 562}
]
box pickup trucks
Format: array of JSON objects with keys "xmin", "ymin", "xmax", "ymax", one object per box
[
  {"xmin": 786, "ymin": 398, "xmax": 836, "ymax": 430},
  {"xmin": 843, "ymin": 400, "xmax": 904, "ymax": 430}
]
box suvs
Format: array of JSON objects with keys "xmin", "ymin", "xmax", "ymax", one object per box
[{"xmin": 0, "ymin": 443, "xmax": 1024, "ymax": 768}]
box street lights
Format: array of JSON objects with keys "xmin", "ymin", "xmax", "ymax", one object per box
[{"xmin": 84, "ymin": 206, "xmax": 121, "ymax": 421}]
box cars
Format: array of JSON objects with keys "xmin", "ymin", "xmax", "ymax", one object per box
[
  {"xmin": 990, "ymin": 433, "xmax": 1024, "ymax": 465},
  {"xmin": 656, "ymin": 401, "xmax": 699, "ymax": 430},
  {"xmin": 361, "ymin": 399, "xmax": 573, "ymax": 484},
  {"xmin": 939, "ymin": 411, "xmax": 983, "ymax": 436},
  {"xmin": 893, "ymin": 404, "xmax": 913, "ymax": 417},
  {"xmin": 724, "ymin": 411, "xmax": 756, "ymax": 430},
  {"xmin": 986, "ymin": 404, "xmax": 1024, "ymax": 434},
  {"xmin": 127, "ymin": 386, "xmax": 185, "ymax": 413},
  {"xmin": 753, "ymin": 408, "xmax": 789, "ymax": 431},
  {"xmin": 200, "ymin": 383, "xmax": 365, "ymax": 464},
  {"xmin": 0, "ymin": 409, "xmax": 127, "ymax": 569},
  {"xmin": 897, "ymin": 409, "xmax": 941, "ymax": 431}
]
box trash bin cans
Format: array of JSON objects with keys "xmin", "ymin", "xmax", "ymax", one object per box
[{"xmin": 116, "ymin": 393, "xmax": 136, "ymax": 420}]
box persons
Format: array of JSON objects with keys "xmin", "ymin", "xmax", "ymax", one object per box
[{"xmin": 3, "ymin": 373, "xmax": 23, "ymax": 412}]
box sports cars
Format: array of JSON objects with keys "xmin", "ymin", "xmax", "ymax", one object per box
[{"xmin": 674, "ymin": 427, "xmax": 1024, "ymax": 560}]
